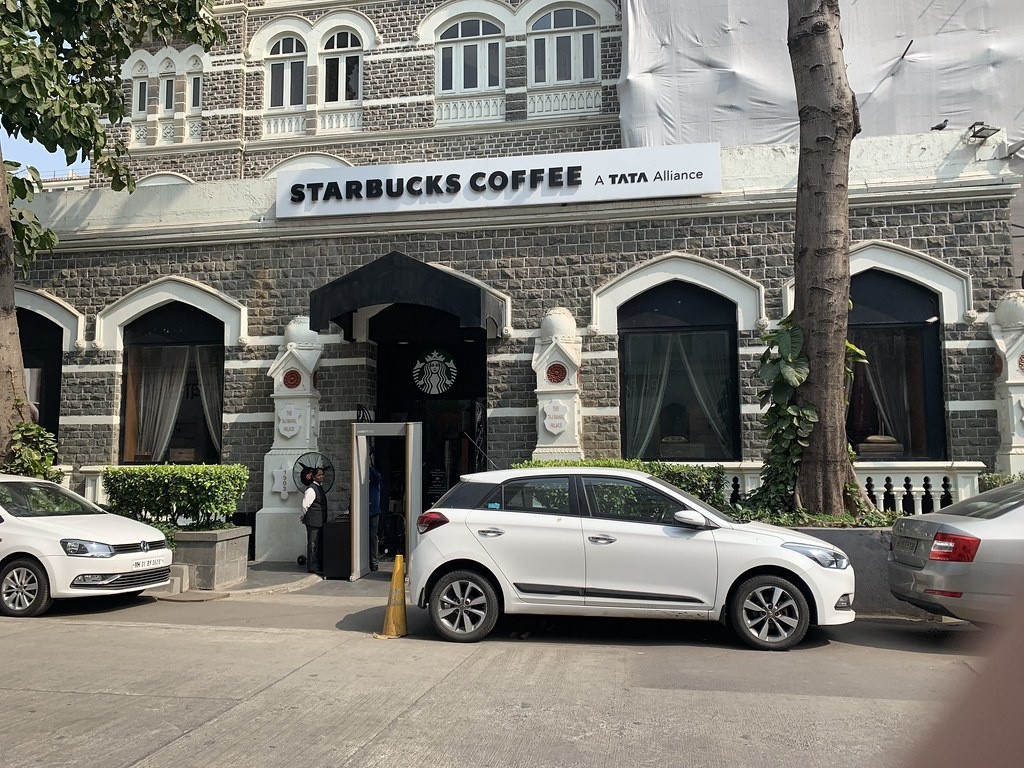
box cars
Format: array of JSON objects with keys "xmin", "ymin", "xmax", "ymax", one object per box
[
  {"xmin": 407, "ymin": 467, "xmax": 859, "ymax": 649},
  {"xmin": 889, "ymin": 477, "xmax": 1024, "ymax": 634},
  {"xmin": 0, "ymin": 473, "xmax": 173, "ymax": 619}
]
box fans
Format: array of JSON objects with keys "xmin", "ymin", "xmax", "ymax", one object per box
[{"xmin": 293, "ymin": 452, "xmax": 336, "ymax": 495}]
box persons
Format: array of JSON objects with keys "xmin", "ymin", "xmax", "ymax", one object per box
[
  {"xmin": 348, "ymin": 461, "xmax": 382, "ymax": 571},
  {"xmin": 298, "ymin": 466, "xmax": 328, "ymax": 575}
]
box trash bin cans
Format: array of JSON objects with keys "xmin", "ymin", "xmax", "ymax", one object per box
[{"xmin": 322, "ymin": 514, "xmax": 351, "ymax": 580}]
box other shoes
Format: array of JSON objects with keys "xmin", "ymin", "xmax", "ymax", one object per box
[
  {"xmin": 309, "ymin": 568, "xmax": 324, "ymax": 574},
  {"xmin": 371, "ymin": 566, "xmax": 378, "ymax": 571}
]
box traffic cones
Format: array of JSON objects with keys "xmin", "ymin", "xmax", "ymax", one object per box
[{"xmin": 377, "ymin": 553, "xmax": 410, "ymax": 641}]
